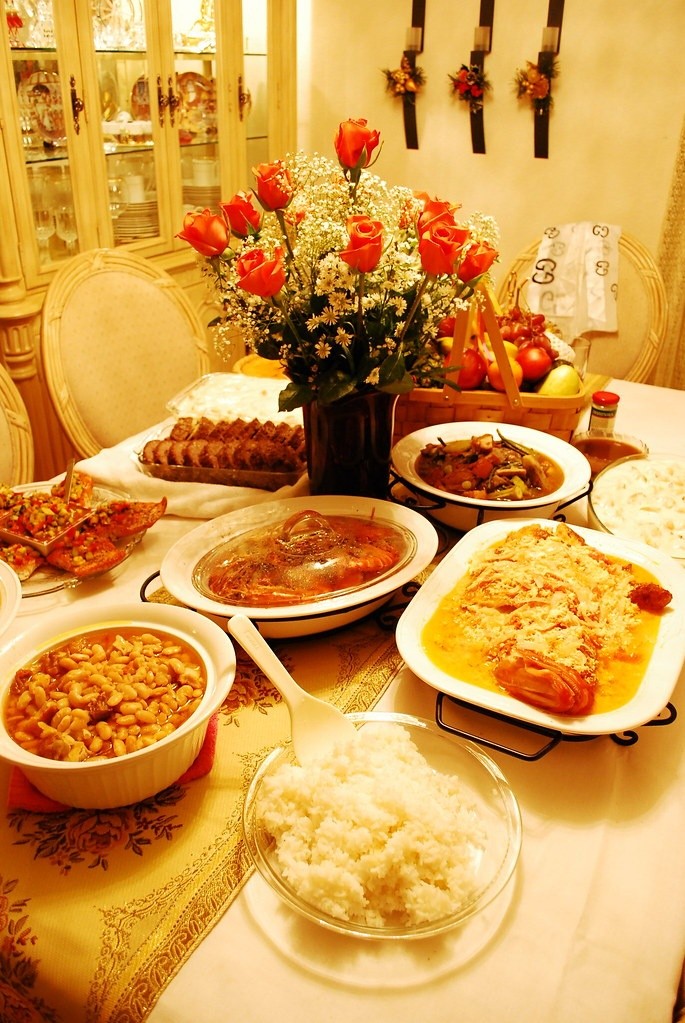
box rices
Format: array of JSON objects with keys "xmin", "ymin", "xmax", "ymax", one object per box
[{"xmin": 256, "ymin": 727, "xmax": 478, "ymax": 928}]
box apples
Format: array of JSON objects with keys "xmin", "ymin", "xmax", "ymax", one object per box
[{"xmin": 434, "ymin": 314, "xmax": 549, "ymax": 391}]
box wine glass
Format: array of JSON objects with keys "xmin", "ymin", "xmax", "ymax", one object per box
[{"xmin": 29, "ymin": 168, "xmax": 78, "ymax": 265}]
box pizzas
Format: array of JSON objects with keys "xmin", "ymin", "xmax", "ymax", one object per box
[{"xmin": 0, "ymin": 469, "xmax": 169, "ymax": 581}]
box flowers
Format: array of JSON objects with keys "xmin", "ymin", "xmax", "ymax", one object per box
[
  {"xmin": 447, "ymin": 63, "xmax": 493, "ymax": 114},
  {"xmin": 508, "ymin": 59, "xmax": 562, "ymax": 108},
  {"xmin": 379, "ymin": 55, "xmax": 428, "ymax": 98},
  {"xmin": 174, "ymin": 118, "xmax": 502, "ymax": 412}
]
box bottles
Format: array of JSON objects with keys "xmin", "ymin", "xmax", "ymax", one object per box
[{"xmin": 587, "ymin": 390, "xmax": 620, "ymax": 439}]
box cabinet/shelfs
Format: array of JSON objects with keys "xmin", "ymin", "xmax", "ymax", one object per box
[{"xmin": 0, "ymin": 0, "xmax": 298, "ymax": 482}]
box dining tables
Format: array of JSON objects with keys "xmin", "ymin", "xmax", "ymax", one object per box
[{"xmin": 0, "ymin": 379, "xmax": 685, "ymax": 1023}]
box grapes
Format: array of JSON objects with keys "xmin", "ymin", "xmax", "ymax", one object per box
[{"xmin": 497, "ymin": 307, "xmax": 560, "ymax": 361}]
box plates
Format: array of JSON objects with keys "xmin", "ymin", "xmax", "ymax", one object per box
[
  {"xmin": 129, "ymin": 372, "xmax": 306, "ymax": 486},
  {"xmin": 181, "ymin": 179, "xmax": 223, "ymax": 218},
  {"xmin": 390, "ymin": 421, "xmax": 591, "ymax": 509},
  {"xmin": 1, "ymin": 481, "xmax": 143, "ymax": 595},
  {"xmin": 394, "ymin": 518, "xmax": 685, "ymax": 736},
  {"xmin": 111, "ymin": 189, "xmax": 158, "ymax": 243}
]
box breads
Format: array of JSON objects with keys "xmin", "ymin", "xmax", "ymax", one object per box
[{"xmin": 142, "ymin": 416, "xmax": 306, "ymax": 491}]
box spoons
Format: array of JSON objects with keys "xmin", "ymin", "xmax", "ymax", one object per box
[{"xmin": 227, "ymin": 613, "xmax": 357, "ymax": 767}]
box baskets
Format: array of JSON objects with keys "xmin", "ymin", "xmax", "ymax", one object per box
[{"xmin": 392, "ymin": 281, "xmax": 585, "ymax": 442}]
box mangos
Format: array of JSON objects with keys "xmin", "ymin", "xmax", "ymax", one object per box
[{"xmin": 532, "ymin": 365, "xmax": 580, "ymax": 397}]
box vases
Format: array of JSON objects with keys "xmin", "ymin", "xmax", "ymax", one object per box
[{"xmin": 301, "ymin": 381, "xmax": 400, "ymax": 498}]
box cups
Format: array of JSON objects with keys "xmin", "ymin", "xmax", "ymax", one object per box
[{"xmin": 570, "ymin": 430, "xmax": 649, "ymax": 481}]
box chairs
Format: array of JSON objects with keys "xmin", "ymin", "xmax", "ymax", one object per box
[
  {"xmin": 39, "ymin": 248, "xmax": 210, "ymax": 459},
  {"xmin": 496, "ymin": 221, "xmax": 669, "ymax": 382},
  {"xmin": 0, "ymin": 362, "xmax": 35, "ymax": 488}
]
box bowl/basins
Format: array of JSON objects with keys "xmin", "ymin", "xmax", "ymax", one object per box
[
  {"xmin": 159, "ymin": 494, "xmax": 439, "ymax": 639},
  {"xmin": 241, "ymin": 710, "xmax": 523, "ymax": 942},
  {"xmin": 0, "ymin": 599, "xmax": 238, "ymax": 809},
  {"xmin": 586, "ymin": 452, "xmax": 685, "ymax": 570}
]
